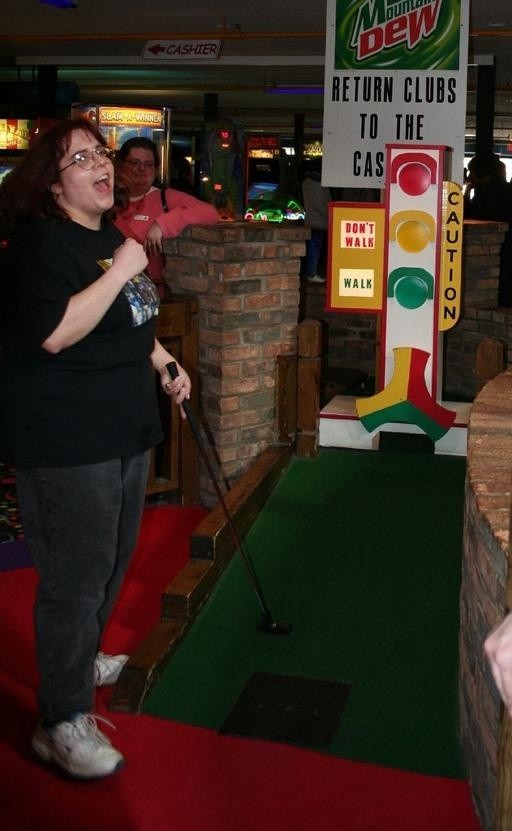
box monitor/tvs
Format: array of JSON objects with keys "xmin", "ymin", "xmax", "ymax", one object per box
[{"xmin": 0, "ymin": 119, "xmax": 30, "ymax": 155}]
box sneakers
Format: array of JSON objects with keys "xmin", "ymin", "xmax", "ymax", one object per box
[
  {"xmin": 308, "ymin": 274, "xmax": 325, "ymax": 282},
  {"xmin": 32, "ymin": 711, "xmax": 126, "ymax": 780},
  {"xmin": 90, "ymin": 652, "xmax": 130, "ymax": 686}
]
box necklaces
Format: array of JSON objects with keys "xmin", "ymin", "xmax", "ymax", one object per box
[{"xmin": 118, "ymin": 192, "xmax": 146, "ymax": 218}]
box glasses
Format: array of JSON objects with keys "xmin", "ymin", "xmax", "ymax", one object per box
[
  {"xmin": 57, "ymin": 145, "xmax": 116, "ymax": 173},
  {"xmin": 123, "ymin": 158, "xmax": 154, "ymax": 167}
]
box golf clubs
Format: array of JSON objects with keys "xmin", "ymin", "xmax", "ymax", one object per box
[{"xmin": 162, "ymin": 361, "xmax": 292, "ymax": 636}]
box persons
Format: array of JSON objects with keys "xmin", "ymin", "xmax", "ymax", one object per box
[
  {"xmin": 300, "ymin": 154, "xmax": 334, "ymax": 285},
  {"xmin": 1, "ymin": 111, "xmax": 193, "ymax": 782},
  {"xmin": 115, "ymin": 136, "xmax": 222, "ymax": 304},
  {"xmin": 464, "ymin": 151, "xmax": 512, "ymax": 307}
]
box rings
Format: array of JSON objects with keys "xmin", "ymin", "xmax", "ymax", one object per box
[{"xmin": 165, "ymin": 381, "xmax": 170, "ymax": 389}]
